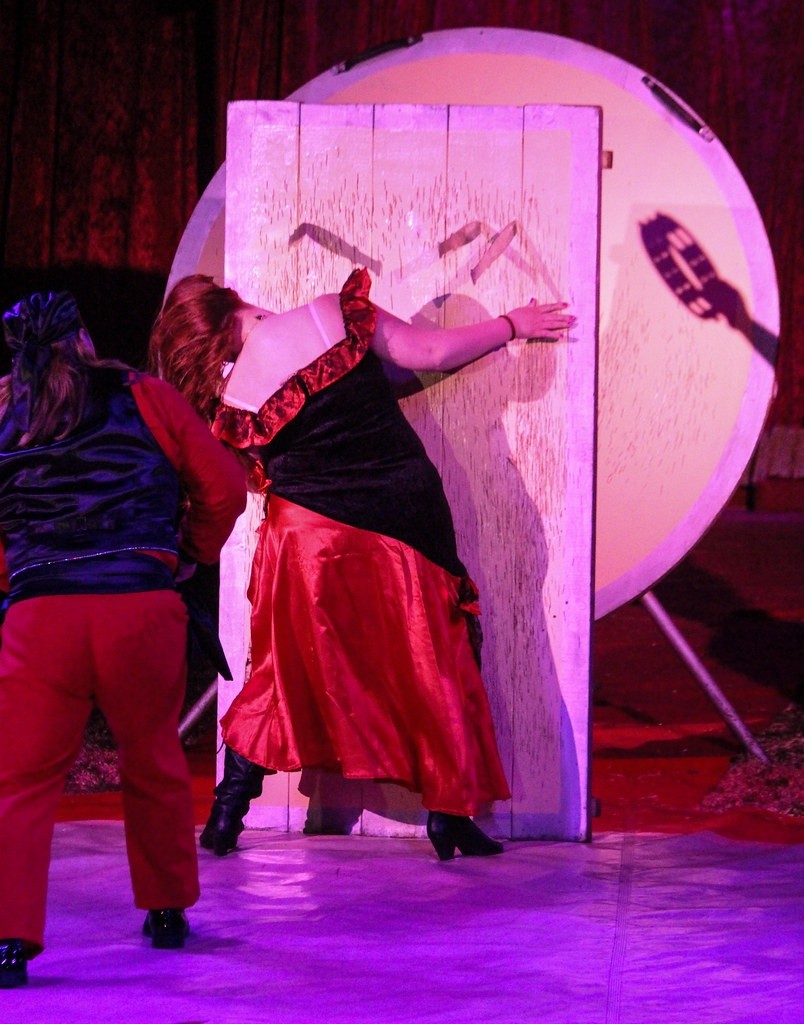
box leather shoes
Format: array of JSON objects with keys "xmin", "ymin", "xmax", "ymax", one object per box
[
  {"xmin": 0, "ymin": 939, "xmax": 29, "ymax": 987},
  {"xmin": 143, "ymin": 908, "xmax": 189, "ymax": 949}
]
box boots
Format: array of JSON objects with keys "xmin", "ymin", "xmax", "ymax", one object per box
[
  {"xmin": 427, "ymin": 811, "xmax": 504, "ymax": 861},
  {"xmin": 198, "ymin": 741, "xmax": 268, "ymax": 856}
]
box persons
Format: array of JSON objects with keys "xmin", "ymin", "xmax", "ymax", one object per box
[
  {"xmin": 151, "ymin": 274, "xmax": 576, "ymax": 862},
  {"xmin": 1, "ymin": 290, "xmax": 248, "ymax": 990}
]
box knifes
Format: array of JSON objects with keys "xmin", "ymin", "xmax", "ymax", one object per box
[
  {"xmin": 471, "ymin": 221, "xmax": 516, "ymax": 286},
  {"xmin": 287, "ymin": 222, "xmax": 306, "ymax": 249},
  {"xmin": 438, "ymin": 220, "xmax": 480, "ymax": 259}
]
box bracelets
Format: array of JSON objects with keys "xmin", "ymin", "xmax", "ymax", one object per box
[{"xmin": 499, "ymin": 310, "xmax": 516, "ymax": 342}]
